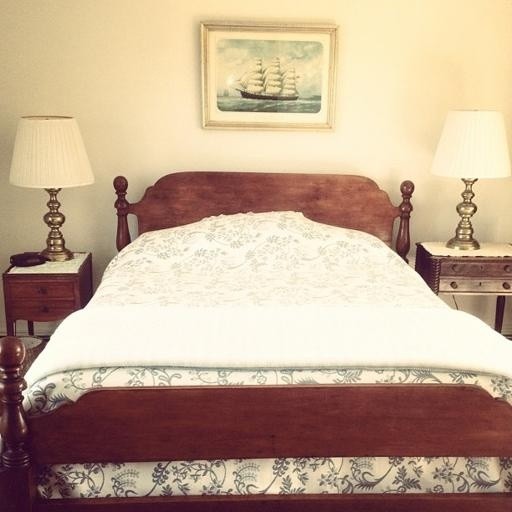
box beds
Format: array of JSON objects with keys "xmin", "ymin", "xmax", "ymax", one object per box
[{"xmin": 0, "ymin": 170, "xmax": 512, "ymax": 511}]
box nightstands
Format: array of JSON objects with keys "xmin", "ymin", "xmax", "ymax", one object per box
[
  {"xmin": 2, "ymin": 251, "xmax": 94, "ymax": 335},
  {"xmin": 415, "ymin": 241, "xmax": 512, "ymax": 334}
]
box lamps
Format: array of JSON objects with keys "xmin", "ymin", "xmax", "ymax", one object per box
[
  {"xmin": 431, "ymin": 110, "xmax": 510, "ymax": 250},
  {"xmin": 9, "ymin": 115, "xmax": 95, "ymax": 261}
]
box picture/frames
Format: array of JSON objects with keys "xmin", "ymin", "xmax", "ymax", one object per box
[{"xmin": 199, "ymin": 19, "xmax": 338, "ymax": 131}]
